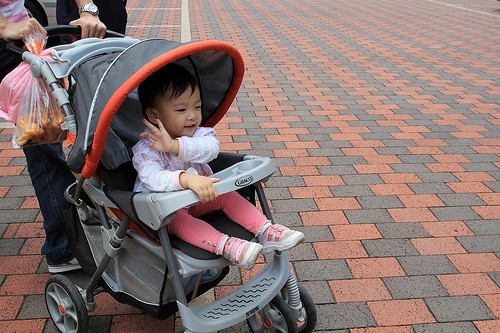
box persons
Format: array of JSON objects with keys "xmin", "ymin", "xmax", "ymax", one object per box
[
  {"xmin": 131, "ymin": 62, "xmax": 306, "ymax": 270},
  {"xmin": 0, "ymin": 0, "xmax": 129, "ymax": 272}
]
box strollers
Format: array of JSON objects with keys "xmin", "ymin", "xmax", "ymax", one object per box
[{"xmin": 6, "ymin": 25, "xmax": 317, "ymax": 333}]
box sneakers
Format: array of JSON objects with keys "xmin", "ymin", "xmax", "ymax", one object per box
[
  {"xmin": 258, "ymin": 223, "xmax": 305, "ymax": 253},
  {"xmin": 224, "ymin": 236, "xmax": 263, "ymax": 270}
]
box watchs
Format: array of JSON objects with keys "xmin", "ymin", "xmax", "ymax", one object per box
[{"xmin": 79, "ymin": 3, "xmax": 99, "ymax": 16}]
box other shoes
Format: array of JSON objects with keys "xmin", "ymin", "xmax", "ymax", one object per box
[{"xmin": 48, "ymin": 257, "xmax": 82, "ymax": 272}]
